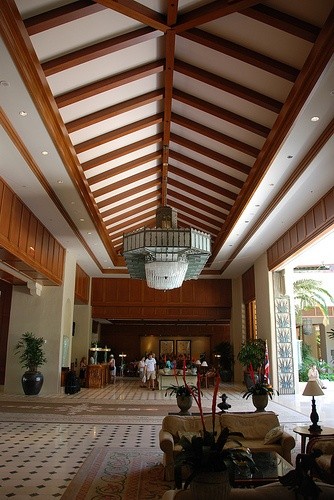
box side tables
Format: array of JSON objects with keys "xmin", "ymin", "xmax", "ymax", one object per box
[{"xmin": 293, "ymin": 426, "xmax": 334, "ymax": 453}]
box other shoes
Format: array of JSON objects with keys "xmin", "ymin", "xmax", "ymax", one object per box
[
  {"xmin": 152, "ymin": 387, "xmax": 155, "ymax": 391},
  {"xmin": 321, "ymin": 386, "xmax": 327, "ymax": 389}
]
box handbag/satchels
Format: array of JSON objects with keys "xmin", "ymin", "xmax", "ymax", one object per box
[{"xmin": 109, "ymin": 366, "xmax": 114, "ymax": 371}]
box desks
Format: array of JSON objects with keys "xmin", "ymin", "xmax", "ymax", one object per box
[{"xmin": 157, "ymin": 370, "xmax": 197, "ymax": 390}]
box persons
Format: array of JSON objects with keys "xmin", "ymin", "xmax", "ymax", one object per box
[
  {"xmin": 108, "ymin": 354, "xmax": 117, "ymax": 385},
  {"xmin": 138, "ymin": 356, "xmax": 147, "ymax": 388},
  {"xmin": 78, "ymin": 357, "xmax": 87, "ymax": 388},
  {"xmin": 303, "ymin": 363, "xmax": 325, "ymax": 395},
  {"xmin": 144, "ymin": 353, "xmax": 160, "ymax": 390}
]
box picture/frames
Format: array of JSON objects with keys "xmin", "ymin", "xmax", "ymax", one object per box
[
  {"xmin": 176, "ymin": 340, "xmax": 191, "ymax": 362},
  {"xmin": 159, "ymin": 340, "xmax": 174, "ymax": 356}
]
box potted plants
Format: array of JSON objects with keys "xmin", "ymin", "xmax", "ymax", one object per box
[
  {"xmin": 14, "ymin": 331, "xmax": 47, "ymax": 395},
  {"xmin": 165, "ymin": 338, "xmax": 279, "ymax": 500}
]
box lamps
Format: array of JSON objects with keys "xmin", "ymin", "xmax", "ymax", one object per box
[
  {"xmin": 201, "ymin": 361, "xmax": 208, "ymax": 376},
  {"xmin": 302, "ymin": 381, "xmax": 325, "ymax": 432},
  {"xmin": 123, "ymin": 206, "xmax": 212, "ymax": 290},
  {"xmin": 195, "ymin": 360, "xmax": 201, "ymax": 370}
]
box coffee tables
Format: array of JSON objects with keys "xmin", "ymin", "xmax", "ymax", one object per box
[{"xmin": 174, "ymin": 451, "xmax": 295, "ymax": 489}]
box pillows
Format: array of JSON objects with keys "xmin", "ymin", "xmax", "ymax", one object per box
[
  {"xmin": 177, "ymin": 430, "xmax": 199, "ymax": 443},
  {"xmin": 316, "ymin": 455, "xmax": 332, "ymax": 473},
  {"xmin": 263, "ymin": 426, "xmax": 284, "ymax": 445}
]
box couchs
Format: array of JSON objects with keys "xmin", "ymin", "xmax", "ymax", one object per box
[
  {"xmin": 159, "ymin": 414, "xmax": 295, "ymax": 482},
  {"xmin": 307, "ymin": 437, "xmax": 334, "ymax": 500}
]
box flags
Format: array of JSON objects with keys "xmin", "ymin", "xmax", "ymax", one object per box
[{"xmin": 264, "ymin": 340, "xmax": 269, "ymax": 382}]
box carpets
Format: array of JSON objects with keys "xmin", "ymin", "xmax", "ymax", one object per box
[{"xmin": 60, "ymin": 447, "xmax": 171, "ymax": 500}]
